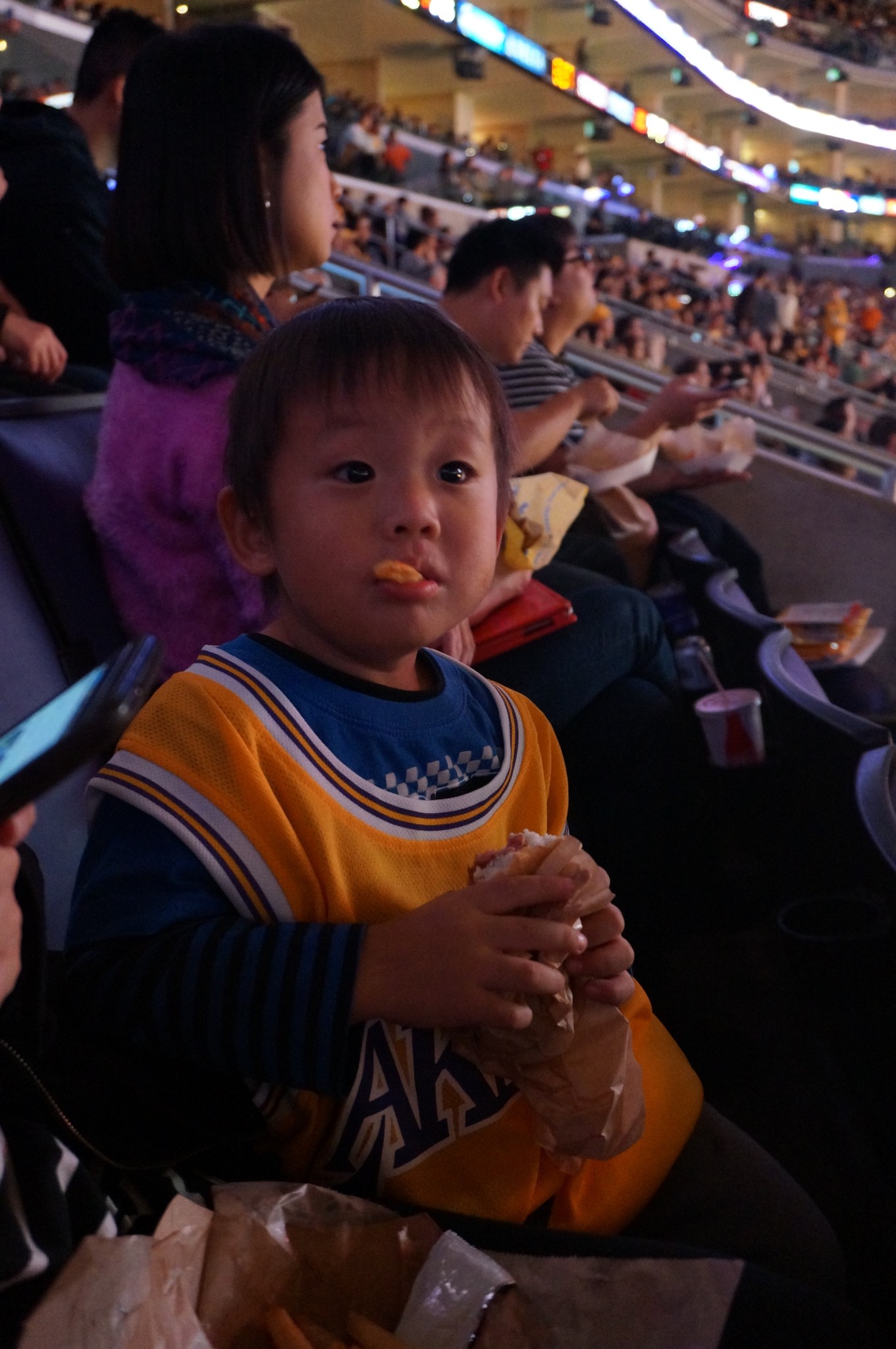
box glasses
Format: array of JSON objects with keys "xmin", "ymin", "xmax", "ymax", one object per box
[{"xmin": 567, "ymin": 250, "xmax": 594, "ymax": 265}]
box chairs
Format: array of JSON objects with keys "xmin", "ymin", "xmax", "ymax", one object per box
[{"xmin": 0, "ymin": 132, "xmax": 896, "ymax": 950}]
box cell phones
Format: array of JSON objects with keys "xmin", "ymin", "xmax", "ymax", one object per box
[
  {"xmin": 718, "ymin": 376, "xmax": 748, "ymax": 393},
  {"xmin": 0, "ymin": 635, "xmax": 166, "ymax": 817}
]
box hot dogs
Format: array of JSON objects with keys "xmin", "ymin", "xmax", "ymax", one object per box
[{"xmin": 467, "ymin": 830, "xmax": 567, "ymax": 886}]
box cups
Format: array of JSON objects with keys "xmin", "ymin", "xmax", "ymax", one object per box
[{"xmin": 697, "ymin": 691, "xmax": 764, "ymax": 768}]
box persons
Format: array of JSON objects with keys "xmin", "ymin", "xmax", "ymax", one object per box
[
  {"xmin": 0, "ymin": 7, "xmax": 182, "ymax": 414},
  {"xmin": 488, "ymin": 212, "xmax": 777, "ymax": 644},
  {"xmin": 58, "ymin": 291, "xmax": 836, "ymax": 1349},
  {"xmin": 76, "ymin": 23, "xmax": 354, "ymax": 691},
  {"xmin": 436, "ymin": 217, "xmax": 631, "ymax": 586},
  {"xmin": 0, "ymin": 799, "xmax": 206, "ymax": 1348},
  {"xmin": 295, "ymin": 73, "xmax": 896, "ymax": 498},
  {"xmin": 0, "ymin": 300, "xmax": 66, "ymax": 384},
  {"xmin": 0, "ymin": 67, "xmax": 74, "ymax": 109}
]
